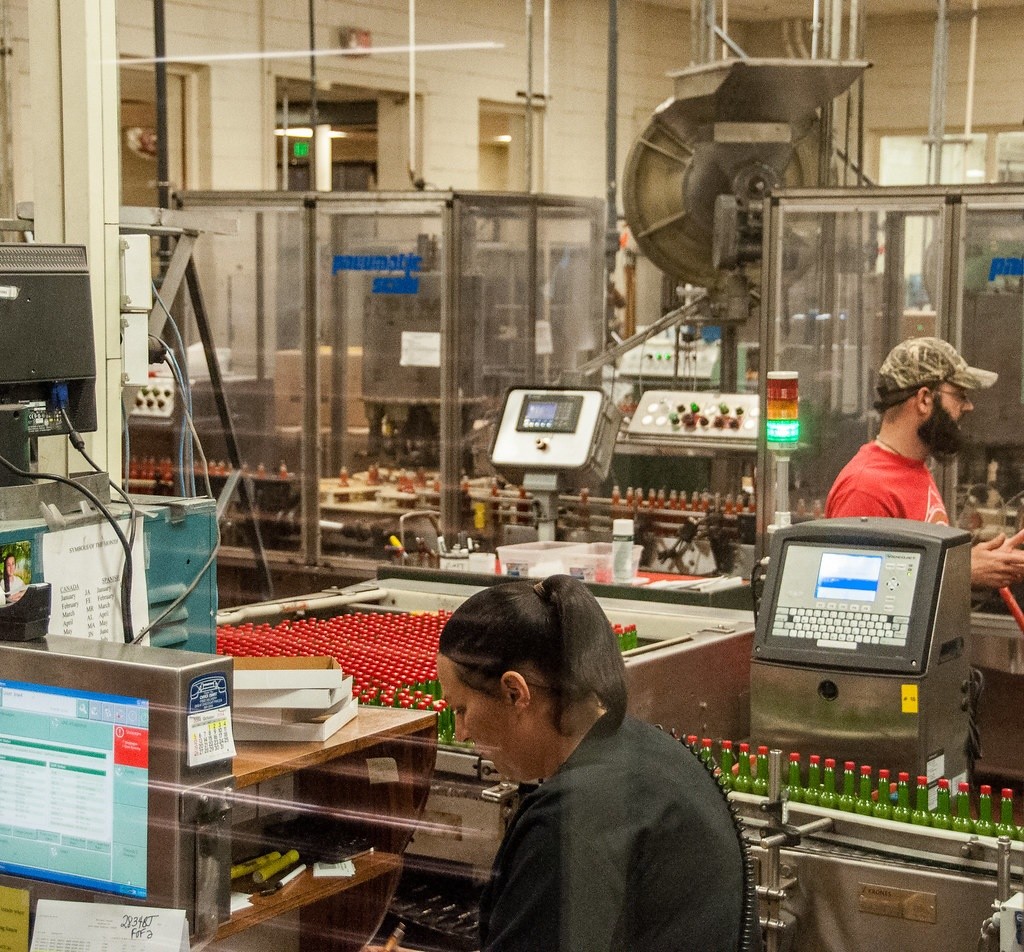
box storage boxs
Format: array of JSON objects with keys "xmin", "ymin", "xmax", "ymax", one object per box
[
  {"xmin": 229, "ymin": 675, "xmax": 354, "ymax": 705},
  {"xmin": 230, "ymin": 693, "xmax": 354, "ymax": 727},
  {"xmin": 232, "ymin": 654, "xmax": 343, "ymax": 689},
  {"xmin": 232, "ymin": 696, "xmax": 360, "ymax": 743}
]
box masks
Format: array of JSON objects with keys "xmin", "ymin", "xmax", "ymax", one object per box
[{"xmin": 916, "ymin": 391, "xmax": 963, "ymax": 465}]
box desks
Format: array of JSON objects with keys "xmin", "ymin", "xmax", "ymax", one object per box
[{"xmin": 170, "ymin": 704, "xmax": 441, "ymax": 952}]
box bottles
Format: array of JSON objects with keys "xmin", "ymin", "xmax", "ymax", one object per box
[
  {"xmin": 194, "ymin": 458, "xmax": 233, "ymax": 497},
  {"xmin": 610, "ymin": 623, "xmax": 637, "ymax": 653},
  {"xmin": 364, "ymin": 458, "xmax": 383, "ymax": 501},
  {"xmin": 580, "ymin": 488, "xmax": 593, "ymax": 532},
  {"xmin": 814, "ymin": 497, "xmax": 822, "ymax": 522},
  {"xmin": 611, "ymin": 484, "xmax": 758, "ymax": 517},
  {"xmin": 689, "ymin": 734, "xmax": 1024, "ymax": 842},
  {"xmin": 130, "ymin": 455, "xmax": 174, "ymax": 495},
  {"xmin": 795, "ymin": 499, "xmax": 808, "ymax": 526},
  {"xmin": 515, "ymin": 485, "xmax": 530, "ymax": 526},
  {"xmin": 216, "ymin": 606, "xmax": 477, "ymax": 749},
  {"xmin": 335, "ymin": 466, "xmax": 350, "ymax": 504},
  {"xmin": 393, "ymin": 464, "xmax": 439, "ymax": 507},
  {"xmin": 459, "ymin": 475, "xmax": 472, "ymax": 530},
  {"xmin": 279, "ymin": 460, "xmax": 288, "ymax": 479},
  {"xmin": 613, "ymin": 518, "xmax": 634, "ymax": 587},
  {"xmin": 486, "ymin": 485, "xmax": 500, "ymax": 526},
  {"xmin": 256, "ymin": 462, "xmax": 265, "ymax": 478}
]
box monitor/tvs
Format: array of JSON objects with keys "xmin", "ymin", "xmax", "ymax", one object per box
[
  {"xmin": 0, "ymin": 679, "xmax": 149, "ymax": 901},
  {"xmin": 763, "ymin": 540, "xmax": 925, "ymax": 660}
]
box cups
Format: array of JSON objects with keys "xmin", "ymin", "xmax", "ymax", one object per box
[{"xmin": 469, "ymin": 552, "xmax": 496, "ymax": 576}]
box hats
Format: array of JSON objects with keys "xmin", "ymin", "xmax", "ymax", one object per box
[{"xmin": 873, "ymin": 336, "xmax": 1000, "ymax": 412}]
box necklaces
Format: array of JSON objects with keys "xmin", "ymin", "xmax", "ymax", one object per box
[{"xmin": 876, "ymin": 434, "xmax": 901, "ymax": 457}]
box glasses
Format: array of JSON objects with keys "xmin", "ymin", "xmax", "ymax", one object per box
[{"xmin": 935, "ymin": 387, "xmax": 976, "ymax": 404}]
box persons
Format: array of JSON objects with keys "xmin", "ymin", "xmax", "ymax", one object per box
[
  {"xmin": 436, "ymin": 575, "xmax": 765, "ymax": 952},
  {"xmin": 1, "ymin": 553, "xmax": 26, "ymax": 603},
  {"xmin": 824, "ymin": 335, "xmax": 1024, "ymax": 592}
]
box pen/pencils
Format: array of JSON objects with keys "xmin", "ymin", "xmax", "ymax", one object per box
[
  {"xmin": 275, "ymin": 864, "xmax": 306, "ymax": 889},
  {"xmin": 384, "ymin": 923, "xmax": 405, "ymax": 952}
]
